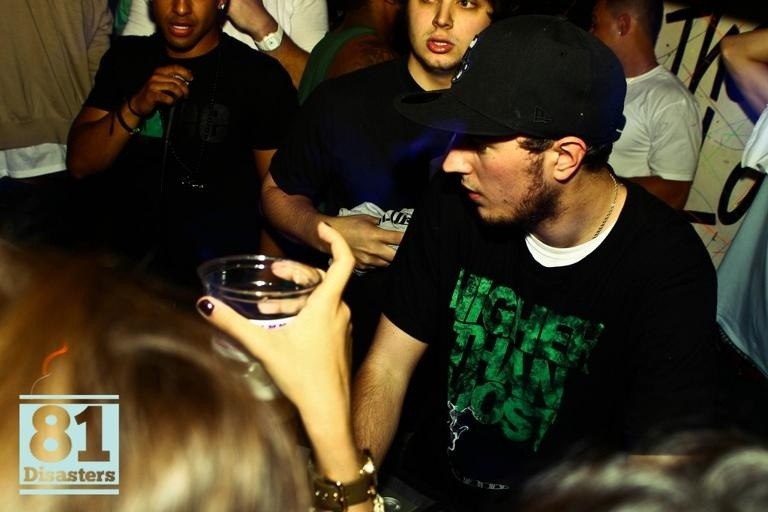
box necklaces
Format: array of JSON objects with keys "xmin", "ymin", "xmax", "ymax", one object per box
[{"xmin": 583, "ymin": 175, "xmax": 619, "ymax": 243}]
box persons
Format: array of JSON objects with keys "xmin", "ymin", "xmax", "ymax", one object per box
[
  {"xmin": 263, "ymin": 0, "xmax": 501, "ymax": 368},
  {"xmin": 295, "ymin": 0, "xmax": 401, "ymax": 106},
  {"xmin": 64, "ymin": 1, "xmax": 296, "ymax": 293},
  {"xmin": 126, "ymin": 0, "xmax": 328, "ymax": 96},
  {"xmin": 719, "ymin": 25, "xmax": 768, "ymax": 430},
  {"xmin": 0, "ymin": 217, "xmax": 382, "ymax": 510},
  {"xmin": 584, "ymin": 2, "xmax": 704, "ymax": 213},
  {"xmin": 351, "ymin": 24, "xmax": 719, "ymax": 511}
]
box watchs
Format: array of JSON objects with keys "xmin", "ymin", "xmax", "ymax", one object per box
[
  {"xmin": 255, "ymin": 25, "xmax": 284, "ymax": 52},
  {"xmin": 311, "ymin": 450, "xmax": 381, "ymax": 512}
]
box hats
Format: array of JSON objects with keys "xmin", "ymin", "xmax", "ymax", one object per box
[{"xmin": 394, "ymin": 13, "xmax": 627, "ymax": 144}]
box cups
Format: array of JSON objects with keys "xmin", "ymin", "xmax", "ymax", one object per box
[{"xmin": 197, "ymin": 253, "xmax": 323, "ymax": 461}]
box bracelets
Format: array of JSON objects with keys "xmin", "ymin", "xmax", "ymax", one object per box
[
  {"xmin": 128, "ymin": 101, "xmax": 146, "ymax": 119},
  {"xmin": 116, "ymin": 108, "xmax": 144, "ymax": 136}
]
